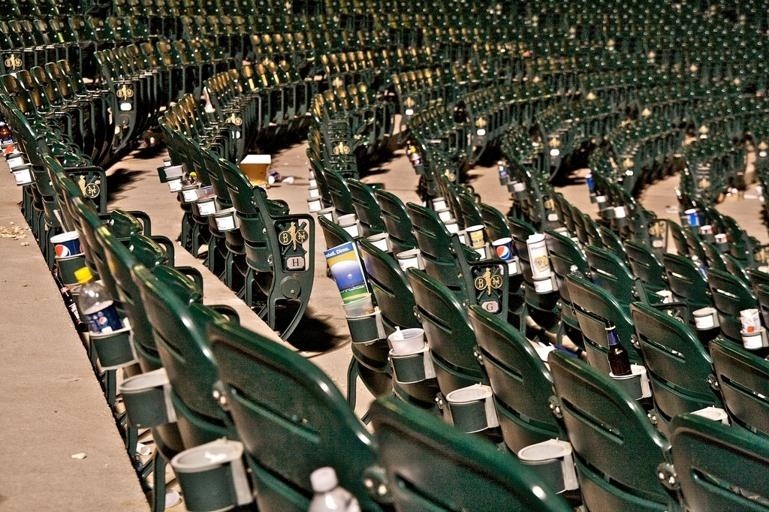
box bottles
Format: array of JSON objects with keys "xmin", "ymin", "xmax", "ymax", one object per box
[
  {"xmin": 691, "ymin": 254, "xmax": 710, "ymax": 284},
  {"xmin": 605, "ymin": 320, "xmax": 632, "ymax": 377},
  {"xmin": 307, "ymin": 466, "xmax": 362, "ymax": 512},
  {"xmin": 74, "ymin": 266, "xmax": 126, "ymax": 336},
  {"xmin": 497, "ymin": 160, "xmax": 507, "ymax": 176},
  {"xmin": 403, "ymin": 139, "xmax": 423, "ymax": 166}
]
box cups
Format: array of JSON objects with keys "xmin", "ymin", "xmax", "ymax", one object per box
[
  {"xmin": 465, "ymin": 224, "xmax": 487, "ymax": 249},
  {"xmin": 50, "ymin": 230, "xmax": 81, "ymax": 258},
  {"xmin": 682, "ymin": 207, "xmax": 703, "ymax": 227},
  {"xmin": 388, "ymin": 327, "xmax": 424, "ymax": 355},
  {"xmin": 492, "ymin": 237, "xmax": 514, "ymax": 262}
]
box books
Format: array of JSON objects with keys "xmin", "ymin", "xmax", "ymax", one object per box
[
  {"xmin": 322, "ymin": 241, "xmax": 370, "ymax": 299},
  {"xmin": 529, "ymin": 233, "xmax": 552, "ymax": 280},
  {"xmin": 740, "ymin": 309, "xmax": 759, "ymax": 333}
]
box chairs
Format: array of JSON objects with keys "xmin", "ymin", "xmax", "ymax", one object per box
[{"xmin": 0, "ymin": 1, "xmax": 767, "ymax": 512}]
